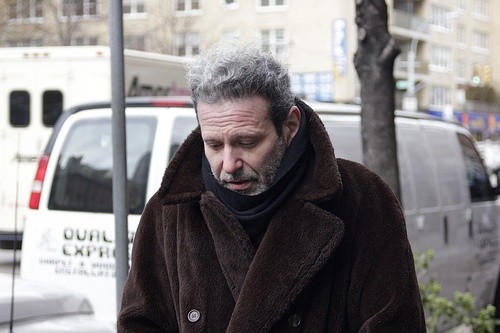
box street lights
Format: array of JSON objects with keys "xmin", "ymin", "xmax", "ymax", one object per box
[{"xmin": 403, "ymin": 12, "xmax": 456, "ymax": 109}]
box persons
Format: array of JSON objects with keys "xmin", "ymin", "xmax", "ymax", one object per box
[{"xmin": 116, "ymin": 41, "xmax": 426, "ymax": 333}]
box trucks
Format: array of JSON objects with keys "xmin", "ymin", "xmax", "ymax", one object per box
[{"xmin": 0, "ymin": 47, "xmax": 198, "ymax": 251}]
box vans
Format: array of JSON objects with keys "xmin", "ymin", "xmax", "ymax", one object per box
[{"xmin": 17, "ymin": 98, "xmax": 500, "ymax": 333}]
